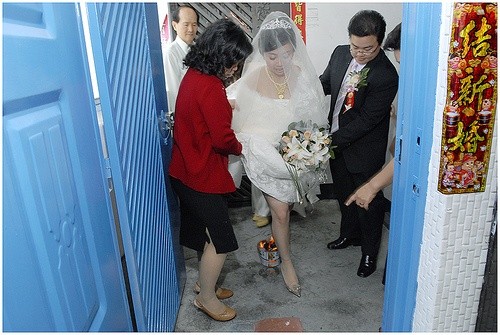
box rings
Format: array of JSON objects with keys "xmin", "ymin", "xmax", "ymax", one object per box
[{"xmin": 360, "ymin": 203, "xmax": 364, "ymax": 205}]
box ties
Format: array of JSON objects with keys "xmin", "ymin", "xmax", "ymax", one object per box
[{"xmin": 330, "ymin": 63, "xmax": 358, "ymax": 133}]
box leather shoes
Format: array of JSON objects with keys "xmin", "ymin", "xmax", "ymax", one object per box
[
  {"xmin": 194, "ymin": 282, "xmax": 233, "ymax": 299},
  {"xmin": 327, "ymin": 236, "xmax": 361, "ymax": 250},
  {"xmin": 194, "ymin": 296, "xmax": 237, "ymax": 321},
  {"xmin": 357, "ymin": 255, "xmax": 376, "ymax": 278}
]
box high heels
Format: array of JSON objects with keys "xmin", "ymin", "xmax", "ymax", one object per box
[{"xmin": 280, "ymin": 263, "xmax": 301, "ymax": 298}]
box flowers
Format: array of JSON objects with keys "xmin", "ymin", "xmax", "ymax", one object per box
[
  {"xmin": 343, "ymin": 67, "xmax": 370, "ymax": 92},
  {"xmin": 275, "ymin": 120, "xmax": 338, "ymax": 171}
]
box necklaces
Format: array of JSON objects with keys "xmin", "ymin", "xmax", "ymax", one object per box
[{"xmin": 266, "ymin": 64, "xmax": 292, "ymax": 99}]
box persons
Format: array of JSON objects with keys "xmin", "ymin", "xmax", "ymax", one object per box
[
  {"xmin": 346, "ymin": 22, "xmax": 402, "ymax": 286},
  {"xmin": 318, "ymin": 9, "xmax": 398, "ymax": 277},
  {"xmin": 169, "ymin": 19, "xmax": 253, "ymax": 321},
  {"xmin": 225, "ymin": 11, "xmax": 330, "ymax": 298},
  {"xmin": 163, "ymin": 5, "xmax": 199, "ymax": 115}
]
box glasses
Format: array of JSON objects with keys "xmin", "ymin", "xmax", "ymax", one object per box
[{"xmin": 350, "ymin": 44, "xmax": 379, "ymax": 55}]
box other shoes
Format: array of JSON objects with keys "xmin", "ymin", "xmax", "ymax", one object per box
[
  {"xmin": 256, "ymin": 215, "xmax": 273, "ymax": 226},
  {"xmin": 252, "ymin": 210, "xmax": 261, "ymax": 221}
]
